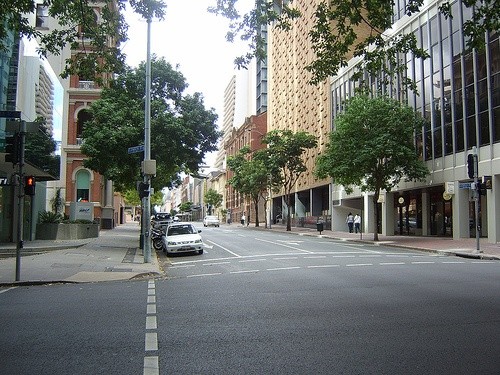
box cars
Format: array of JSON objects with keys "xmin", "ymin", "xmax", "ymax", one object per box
[
  {"xmin": 203, "ymin": 216, "xmax": 220, "ymax": 228},
  {"xmin": 155, "ymin": 213, "xmax": 174, "ymax": 228},
  {"xmin": 162, "ymin": 222, "xmax": 204, "ymax": 255}
]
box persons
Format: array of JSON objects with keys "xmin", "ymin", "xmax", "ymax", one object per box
[
  {"xmin": 346, "ymin": 213, "xmax": 362, "ymax": 234},
  {"xmin": 240, "ymin": 212, "xmax": 251, "ymax": 228}
]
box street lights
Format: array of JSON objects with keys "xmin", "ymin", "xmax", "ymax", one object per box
[{"xmin": 248, "ymin": 129, "xmax": 272, "ymax": 229}]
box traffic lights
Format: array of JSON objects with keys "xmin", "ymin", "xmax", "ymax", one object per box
[
  {"xmin": 467, "ymin": 154, "xmax": 475, "ymax": 178},
  {"xmin": 26, "ymin": 176, "xmax": 35, "ymax": 195},
  {"xmin": 138, "ymin": 182, "xmax": 149, "ymax": 198},
  {"xmin": 4, "ymin": 132, "xmax": 19, "ymax": 165}
]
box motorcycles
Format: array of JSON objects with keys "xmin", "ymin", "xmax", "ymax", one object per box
[{"xmin": 151, "ymin": 228, "xmax": 162, "ymax": 250}]
